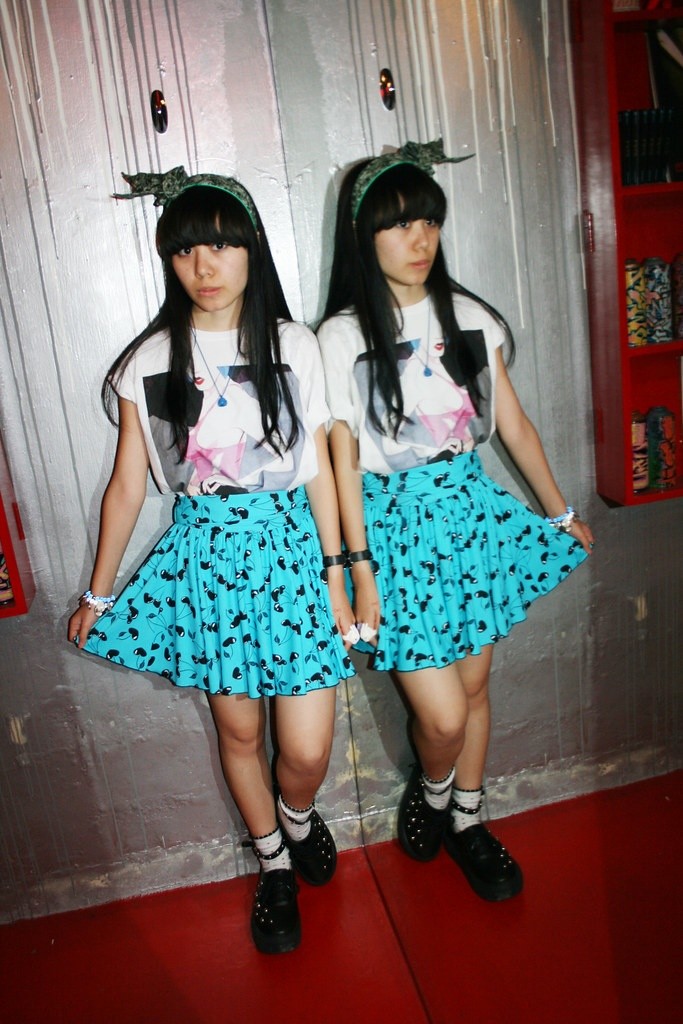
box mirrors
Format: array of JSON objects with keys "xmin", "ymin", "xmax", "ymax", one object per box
[{"xmin": 261, "ymin": 1, "xmax": 682, "ymax": 1024}]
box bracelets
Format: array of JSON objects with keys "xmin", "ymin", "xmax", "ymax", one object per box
[
  {"xmin": 348, "ymin": 549, "xmax": 373, "ymax": 564},
  {"xmin": 80, "ymin": 591, "xmax": 115, "ymax": 615},
  {"xmin": 545, "ymin": 507, "xmax": 574, "ymax": 530},
  {"xmin": 322, "ymin": 554, "xmax": 347, "ymax": 567}
]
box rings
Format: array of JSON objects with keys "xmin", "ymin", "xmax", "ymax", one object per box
[
  {"xmin": 358, "ymin": 622, "xmax": 376, "ymax": 642},
  {"xmin": 342, "ymin": 625, "xmax": 360, "ymax": 645}
]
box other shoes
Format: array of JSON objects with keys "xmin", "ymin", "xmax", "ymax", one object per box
[
  {"xmin": 398, "ymin": 758, "xmax": 453, "ymax": 861},
  {"xmin": 241, "ymin": 838, "xmax": 302, "ymax": 955},
  {"xmin": 442, "ymin": 800, "xmax": 524, "ymax": 901},
  {"xmin": 274, "ymin": 802, "xmax": 337, "ymax": 886}
]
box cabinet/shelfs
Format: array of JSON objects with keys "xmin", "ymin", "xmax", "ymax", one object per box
[{"xmin": 572, "ymin": 1, "xmax": 683, "ymax": 506}]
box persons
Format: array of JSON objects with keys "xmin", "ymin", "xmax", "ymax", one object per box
[
  {"xmin": 66, "ymin": 166, "xmax": 358, "ymax": 954},
  {"xmin": 318, "ymin": 136, "xmax": 594, "ymax": 906}
]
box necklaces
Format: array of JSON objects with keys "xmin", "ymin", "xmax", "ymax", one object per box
[
  {"xmin": 390, "ymin": 298, "xmax": 432, "ymax": 376},
  {"xmin": 188, "ymin": 325, "xmax": 241, "ymax": 407}
]
